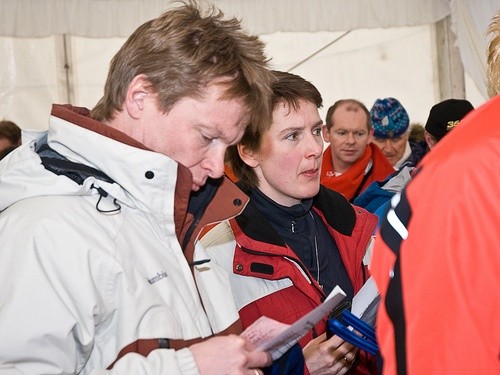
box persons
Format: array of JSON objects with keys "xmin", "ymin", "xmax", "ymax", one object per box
[{"xmin": 0, "ymin": 0, "xmax": 500, "ymax": 375}]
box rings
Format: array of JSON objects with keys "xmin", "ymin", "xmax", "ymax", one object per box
[
  {"xmin": 253, "ymin": 368, "xmax": 259, "ymax": 375},
  {"xmin": 343, "ymin": 356, "xmax": 348, "ymax": 362}
]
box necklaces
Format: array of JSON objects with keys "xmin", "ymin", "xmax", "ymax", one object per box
[{"xmin": 308, "ymin": 211, "xmax": 323, "ymax": 290}]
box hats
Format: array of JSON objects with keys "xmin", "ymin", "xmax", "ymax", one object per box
[
  {"xmin": 425, "ymin": 99, "xmax": 474, "ymax": 143},
  {"xmin": 369, "ymin": 98, "xmax": 409, "ymax": 139}
]
